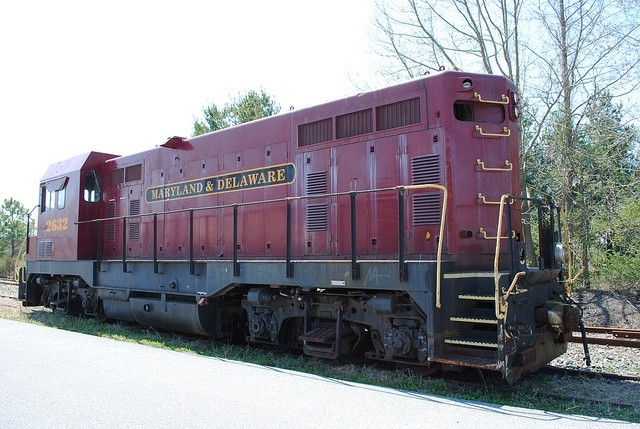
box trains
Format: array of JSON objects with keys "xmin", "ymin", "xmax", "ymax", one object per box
[{"xmin": 19, "ymin": 66, "xmax": 590, "ymax": 388}]
